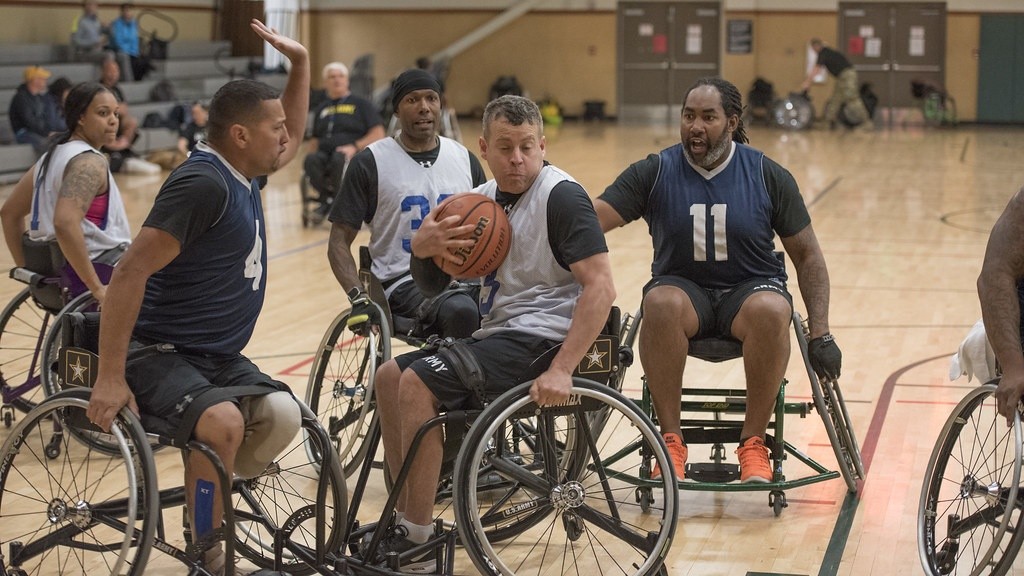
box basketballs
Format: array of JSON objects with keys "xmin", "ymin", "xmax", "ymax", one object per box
[{"xmin": 432, "ymin": 193, "xmax": 509, "ymax": 277}]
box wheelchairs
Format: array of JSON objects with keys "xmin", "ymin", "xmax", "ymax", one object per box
[
  {"xmin": 0, "ymin": 229, "xmax": 184, "ymax": 461},
  {"xmin": 0, "ymin": 311, "xmax": 348, "ymax": 576},
  {"xmin": 303, "ymin": 246, "xmax": 679, "ymax": 576},
  {"xmin": 917, "ymin": 373, "xmax": 1024, "ymax": 576},
  {"xmin": 579, "ymin": 252, "xmax": 863, "ymax": 516},
  {"xmin": 770, "ymin": 91, "xmax": 813, "ymax": 132}
]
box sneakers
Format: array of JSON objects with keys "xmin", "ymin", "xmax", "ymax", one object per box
[
  {"xmin": 650, "ymin": 432, "xmax": 688, "ymax": 482},
  {"xmin": 184, "ymin": 545, "xmax": 245, "ymax": 576},
  {"xmin": 734, "ymin": 436, "xmax": 774, "ymax": 484},
  {"xmin": 352, "ymin": 510, "xmax": 419, "ymax": 564}
]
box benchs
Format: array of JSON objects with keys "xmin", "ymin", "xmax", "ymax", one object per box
[{"xmin": 0, "ymin": 42, "xmax": 314, "ymax": 187}]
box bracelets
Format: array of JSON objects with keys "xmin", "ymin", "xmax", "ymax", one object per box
[{"xmin": 352, "ymin": 142, "xmax": 359, "ymax": 153}]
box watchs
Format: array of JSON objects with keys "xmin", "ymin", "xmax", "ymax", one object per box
[
  {"xmin": 186, "ymin": 151, "xmax": 192, "ymax": 158},
  {"xmin": 346, "ymin": 286, "xmax": 367, "ymax": 302}
]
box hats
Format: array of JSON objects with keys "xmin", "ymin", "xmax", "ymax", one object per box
[
  {"xmin": 392, "ymin": 68, "xmax": 442, "ymax": 112},
  {"xmin": 24, "ymin": 65, "xmax": 51, "ymax": 82}
]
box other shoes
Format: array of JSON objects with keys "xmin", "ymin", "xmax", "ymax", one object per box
[{"xmin": 310, "ymin": 203, "xmax": 331, "ymax": 222}]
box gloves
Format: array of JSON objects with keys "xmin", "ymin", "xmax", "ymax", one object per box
[{"xmin": 808, "ymin": 332, "xmax": 842, "ymax": 383}]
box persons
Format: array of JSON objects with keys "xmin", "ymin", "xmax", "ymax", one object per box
[
  {"xmin": 304, "ymin": 62, "xmax": 386, "ymax": 224},
  {"xmin": 416, "ymin": 57, "xmax": 446, "ymax": 107},
  {"xmin": 8, "ymin": 0, "xmax": 155, "ymax": 173},
  {"xmin": 176, "ymin": 100, "xmax": 209, "ymax": 159},
  {"xmin": 0, "ymin": 82, "xmax": 134, "ymax": 308},
  {"xmin": 977, "ymin": 182, "xmax": 1024, "ymax": 427},
  {"xmin": 85, "ymin": 18, "xmax": 311, "ymax": 576},
  {"xmin": 327, "ymin": 70, "xmax": 522, "ymax": 488},
  {"xmin": 592, "ymin": 78, "xmax": 842, "ymax": 484},
  {"xmin": 351, "ymin": 94, "xmax": 617, "ymax": 566},
  {"xmin": 800, "ymin": 37, "xmax": 875, "ymax": 135}
]
